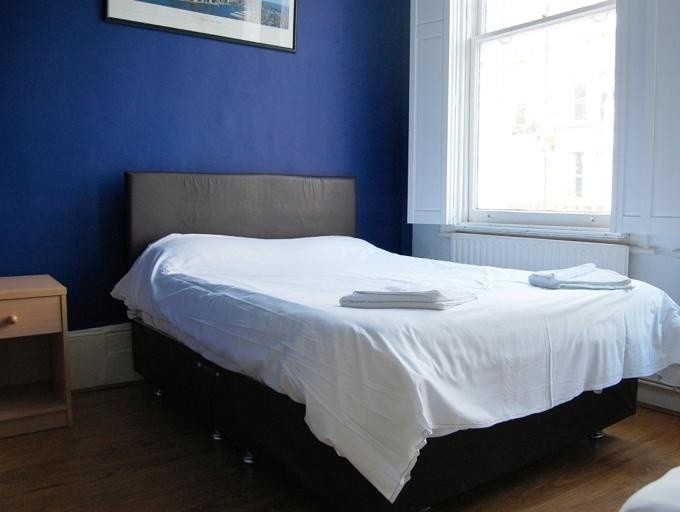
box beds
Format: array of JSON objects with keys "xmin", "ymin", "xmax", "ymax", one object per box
[{"xmin": 123, "ymin": 167, "xmax": 647, "ymax": 512}]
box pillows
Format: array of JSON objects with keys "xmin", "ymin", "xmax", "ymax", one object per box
[{"xmin": 146, "ymin": 232, "xmax": 376, "ymax": 273}]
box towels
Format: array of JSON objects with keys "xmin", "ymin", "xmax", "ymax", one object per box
[
  {"xmin": 338, "ymin": 288, "xmax": 477, "ymax": 310},
  {"xmin": 529, "ymin": 263, "xmax": 635, "ymax": 290}
]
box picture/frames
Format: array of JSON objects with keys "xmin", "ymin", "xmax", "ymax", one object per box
[{"xmin": 103, "ymin": 0, "xmax": 299, "ymax": 53}]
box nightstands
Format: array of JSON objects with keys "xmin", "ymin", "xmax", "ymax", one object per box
[{"xmin": 0, "ymin": 275, "xmax": 74, "ymax": 439}]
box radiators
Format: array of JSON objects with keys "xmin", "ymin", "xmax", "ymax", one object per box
[{"xmin": 449, "ymin": 232, "xmax": 632, "ymax": 276}]
